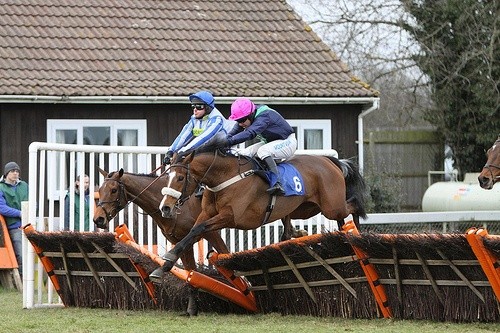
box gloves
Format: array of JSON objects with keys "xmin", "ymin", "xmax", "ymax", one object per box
[{"xmin": 163, "ymin": 151, "xmax": 173, "ymax": 166}]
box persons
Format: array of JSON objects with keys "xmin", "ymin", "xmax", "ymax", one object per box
[
  {"xmin": 163, "ymin": 92, "xmax": 237, "ymax": 197},
  {"xmin": 0, "ymin": 162, "xmax": 28, "ymax": 278},
  {"xmin": 222, "ymin": 97, "xmax": 297, "ymax": 194},
  {"xmin": 64, "ymin": 174, "xmax": 97, "ymax": 231}
]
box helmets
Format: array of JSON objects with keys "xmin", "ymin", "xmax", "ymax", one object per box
[
  {"xmin": 228, "ymin": 98, "xmax": 255, "ymax": 121},
  {"xmin": 190, "ymin": 91, "xmax": 215, "ymax": 109}
]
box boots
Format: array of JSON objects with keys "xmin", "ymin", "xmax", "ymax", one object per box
[{"xmin": 263, "ymin": 156, "xmax": 285, "ymax": 196}]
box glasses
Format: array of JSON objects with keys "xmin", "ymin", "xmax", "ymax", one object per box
[{"xmin": 191, "ymin": 105, "xmax": 206, "ymax": 110}]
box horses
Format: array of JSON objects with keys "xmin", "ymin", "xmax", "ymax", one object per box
[
  {"xmin": 477, "ymin": 135, "xmax": 500, "ymax": 190},
  {"xmin": 93, "ymin": 151, "xmax": 371, "ymax": 315}
]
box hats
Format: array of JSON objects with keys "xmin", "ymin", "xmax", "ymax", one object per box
[{"xmin": 4, "ymin": 162, "xmax": 20, "ymax": 176}]
